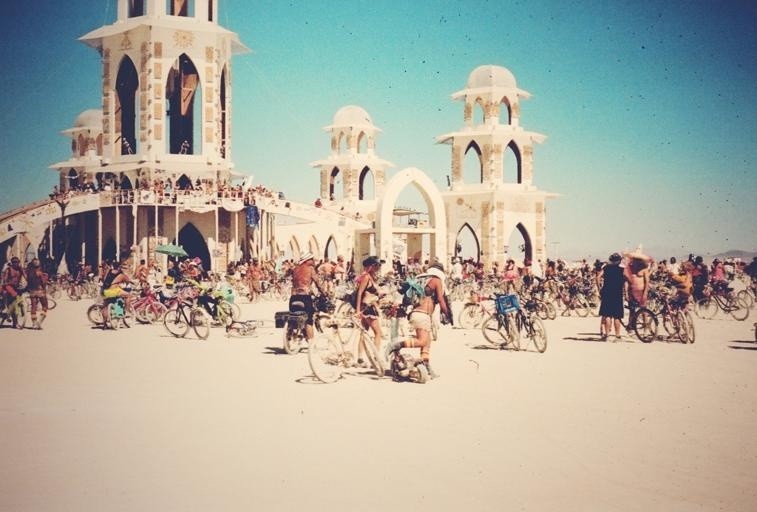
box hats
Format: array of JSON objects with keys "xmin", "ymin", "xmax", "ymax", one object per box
[
  {"xmin": 609, "ymin": 253, "xmax": 622, "ymax": 264},
  {"xmin": 362, "ymin": 256, "xmax": 386, "ymax": 267},
  {"xmin": 299, "ymin": 251, "xmax": 313, "ymax": 265}
]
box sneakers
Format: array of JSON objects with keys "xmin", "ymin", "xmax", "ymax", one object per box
[
  {"xmin": 384, "ymin": 342, "xmax": 393, "ymax": 362},
  {"xmin": 428, "ymin": 371, "xmax": 440, "ymax": 381}
]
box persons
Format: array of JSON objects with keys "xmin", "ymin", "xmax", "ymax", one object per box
[
  {"xmin": 353, "ymin": 255, "xmax": 382, "ymax": 369},
  {"xmin": 383, "ymin": 260, "xmax": 453, "ymax": 380},
  {"xmin": 1, "ymin": 242, "xmax": 757, "ymax": 350}
]
